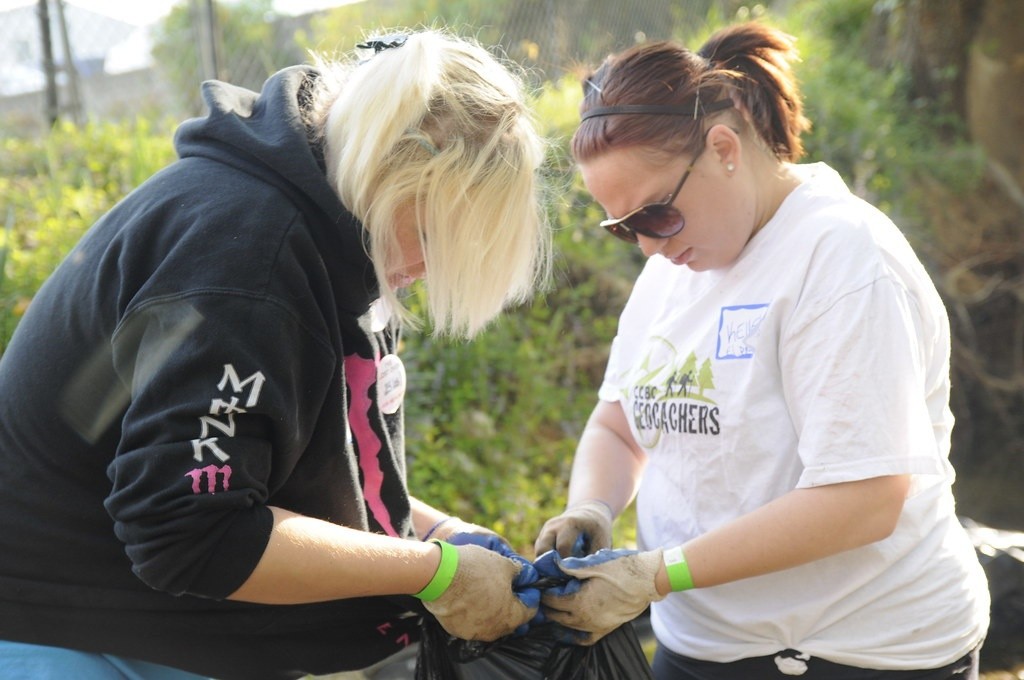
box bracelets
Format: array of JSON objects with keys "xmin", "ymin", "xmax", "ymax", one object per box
[
  {"xmin": 411, "ymin": 539, "xmax": 459, "ymax": 603},
  {"xmin": 662, "ymin": 546, "xmax": 695, "ymax": 592}
]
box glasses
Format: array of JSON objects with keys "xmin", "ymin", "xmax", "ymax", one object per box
[{"xmin": 598, "ymin": 125, "xmax": 740, "ymax": 244}]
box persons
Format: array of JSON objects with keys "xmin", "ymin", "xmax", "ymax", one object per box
[
  {"xmin": 538, "ymin": 24, "xmax": 991, "ymax": 679},
  {"xmin": 0, "ymin": 32, "xmax": 553, "ymax": 680}
]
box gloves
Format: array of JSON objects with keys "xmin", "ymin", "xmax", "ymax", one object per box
[
  {"xmin": 413, "ymin": 537, "xmax": 541, "ymax": 643},
  {"xmin": 424, "ymin": 517, "xmax": 533, "ymax": 564},
  {"xmin": 530, "ymin": 545, "xmax": 667, "ymax": 647},
  {"xmin": 533, "ymin": 500, "xmax": 614, "ymax": 574}
]
